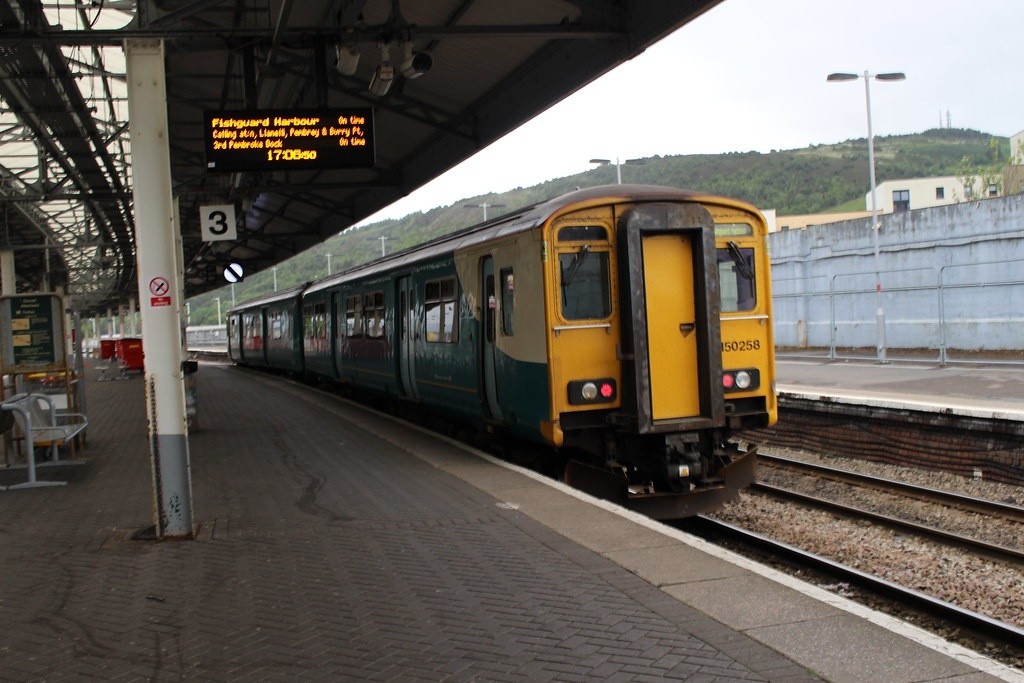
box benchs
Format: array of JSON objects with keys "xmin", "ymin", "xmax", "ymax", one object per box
[
  {"xmin": 115, "ymin": 355, "xmax": 131, "ymax": 380},
  {"xmin": 93, "ymin": 356, "xmax": 113, "ymax": 382},
  {"xmin": 0, "ymin": 392, "xmax": 91, "ymax": 489}
]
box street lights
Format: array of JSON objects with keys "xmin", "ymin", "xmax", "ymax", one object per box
[
  {"xmin": 827, "ymin": 70, "xmax": 906, "ymax": 364},
  {"xmin": 317, "ymin": 252, "xmax": 342, "ymax": 276},
  {"xmin": 589, "ymin": 158, "xmax": 647, "ymax": 185},
  {"xmin": 267, "ymin": 266, "xmax": 285, "ymax": 292},
  {"xmin": 212, "ymin": 297, "xmax": 221, "ymax": 325},
  {"xmin": 463, "ymin": 203, "xmax": 505, "ymax": 221},
  {"xmin": 367, "ymin": 235, "xmax": 399, "ymax": 256}
]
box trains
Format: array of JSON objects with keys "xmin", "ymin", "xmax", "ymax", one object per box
[{"xmin": 225, "ymin": 183, "xmax": 777, "ymax": 520}]
